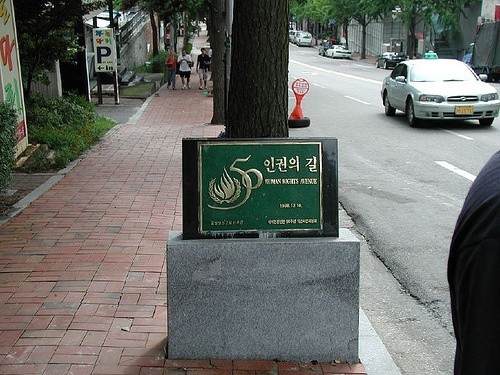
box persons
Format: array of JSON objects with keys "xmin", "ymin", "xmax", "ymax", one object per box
[
  {"xmin": 165, "ymin": 28, "xmax": 171, "ymax": 51},
  {"xmin": 165, "ymin": 50, "xmax": 177, "ymax": 90},
  {"xmin": 447, "ymin": 151, "xmax": 500, "ymax": 375},
  {"xmin": 177, "ymin": 48, "xmax": 192, "ymax": 89},
  {"xmin": 196, "ymin": 47, "xmax": 212, "ymax": 89}
]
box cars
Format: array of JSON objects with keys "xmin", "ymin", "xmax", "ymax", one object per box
[
  {"xmin": 324, "ymin": 44, "xmax": 352, "ymax": 59},
  {"xmin": 375, "ymin": 52, "xmax": 406, "ymax": 69},
  {"xmin": 295, "ymin": 33, "xmax": 313, "ymax": 47},
  {"xmin": 289, "ymin": 30, "xmax": 302, "ymax": 44},
  {"xmin": 380, "ymin": 51, "xmax": 500, "ymax": 127},
  {"xmin": 318, "ymin": 41, "xmax": 340, "ymax": 55}
]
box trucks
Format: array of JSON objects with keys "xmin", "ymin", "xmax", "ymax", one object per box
[{"xmin": 461, "ymin": 21, "xmax": 500, "ymax": 82}]
